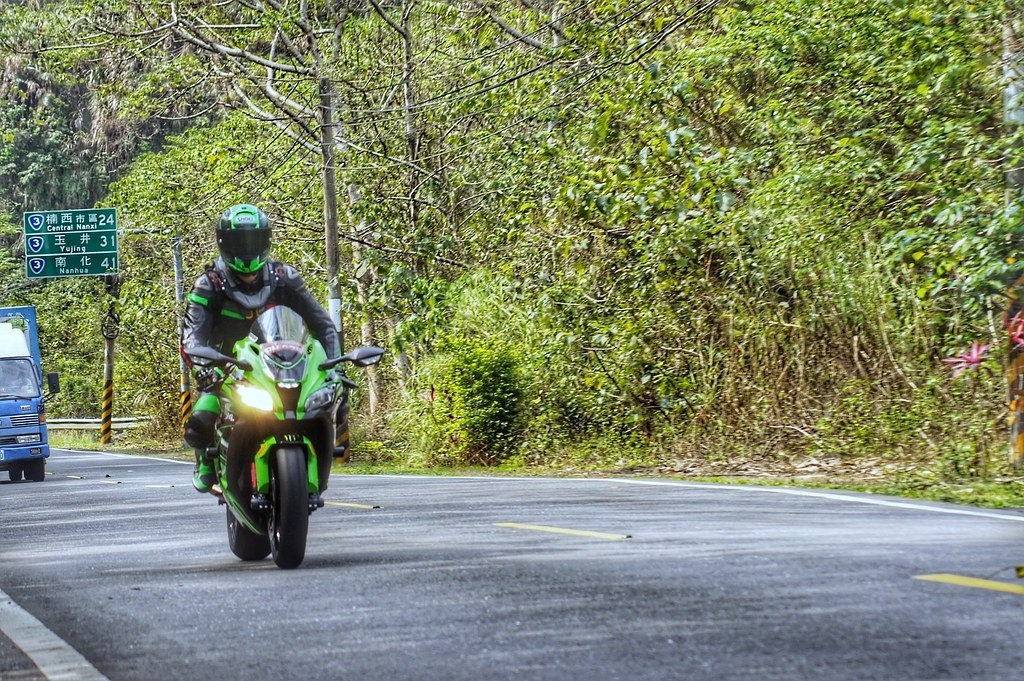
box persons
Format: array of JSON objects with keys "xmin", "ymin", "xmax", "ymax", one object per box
[
  {"xmin": 0, "ymin": 364, "xmax": 34, "ymax": 393},
  {"xmin": 181, "ymin": 203, "xmax": 343, "ymax": 493}
]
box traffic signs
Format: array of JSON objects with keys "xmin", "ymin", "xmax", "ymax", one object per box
[{"xmin": 23, "ymin": 206, "xmax": 119, "ymax": 279}]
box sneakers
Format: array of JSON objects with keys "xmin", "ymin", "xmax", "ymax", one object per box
[{"xmin": 190, "ymin": 448, "xmax": 214, "ymax": 492}]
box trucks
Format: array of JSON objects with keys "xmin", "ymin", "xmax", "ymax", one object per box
[{"xmin": 0, "ymin": 304, "xmax": 61, "ymax": 483}]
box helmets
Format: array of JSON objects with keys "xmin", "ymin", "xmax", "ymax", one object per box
[{"xmin": 215, "ymin": 204, "xmax": 273, "ymax": 275}]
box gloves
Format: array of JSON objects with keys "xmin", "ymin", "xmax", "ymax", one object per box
[
  {"xmin": 325, "ymin": 369, "xmax": 357, "ymax": 390},
  {"xmin": 195, "ymin": 367, "xmax": 221, "ymax": 390}
]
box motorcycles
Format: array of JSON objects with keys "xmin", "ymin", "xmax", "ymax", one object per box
[{"xmin": 183, "ymin": 304, "xmax": 384, "ymax": 570}]
